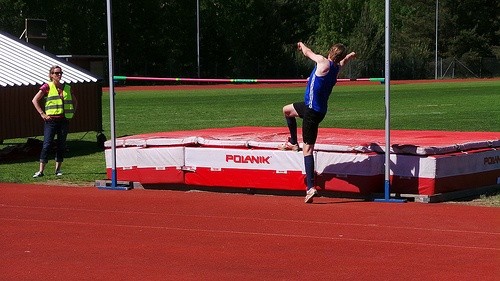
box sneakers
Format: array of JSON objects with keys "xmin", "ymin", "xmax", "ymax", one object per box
[
  {"xmin": 55, "ymin": 170, "xmax": 62, "ymax": 176},
  {"xmin": 33, "ymin": 171, "xmax": 43, "ymax": 178},
  {"xmin": 304, "ymin": 188, "xmax": 317, "ymax": 203},
  {"xmin": 277, "ymin": 136, "xmax": 299, "ymax": 152}
]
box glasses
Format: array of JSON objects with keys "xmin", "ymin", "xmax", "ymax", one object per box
[{"xmin": 52, "ymin": 72, "xmax": 63, "ymax": 75}]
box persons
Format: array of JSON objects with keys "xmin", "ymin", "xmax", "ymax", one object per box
[
  {"xmin": 277, "ymin": 42, "xmax": 356, "ymax": 203},
  {"xmin": 32, "ymin": 66, "xmax": 78, "ymax": 178}
]
